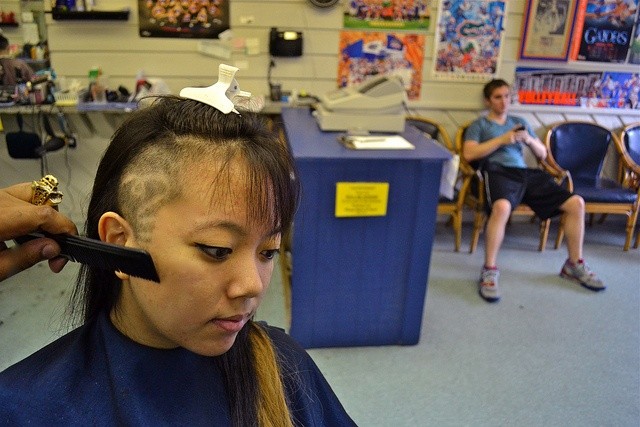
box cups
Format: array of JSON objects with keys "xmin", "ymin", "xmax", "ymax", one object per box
[{"xmin": 89, "ymin": 65, "xmax": 106, "ymax": 103}]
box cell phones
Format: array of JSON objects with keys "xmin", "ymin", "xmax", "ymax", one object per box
[{"xmin": 515, "ymin": 125, "xmax": 526, "ymax": 134}]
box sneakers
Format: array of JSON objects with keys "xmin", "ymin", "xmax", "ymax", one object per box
[
  {"xmin": 560, "ymin": 259, "xmax": 607, "ymax": 291},
  {"xmin": 479, "ymin": 266, "xmax": 500, "ymax": 301}
]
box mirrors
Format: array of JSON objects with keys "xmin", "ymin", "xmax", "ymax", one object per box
[{"xmin": 0, "ymin": 0, "xmax": 51, "ymax": 91}]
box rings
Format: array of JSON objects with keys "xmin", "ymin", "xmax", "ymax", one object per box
[{"xmin": 32, "ymin": 174, "xmax": 64, "ymax": 206}]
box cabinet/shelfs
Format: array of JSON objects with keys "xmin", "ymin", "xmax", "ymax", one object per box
[
  {"xmin": 51, "ymin": 7, "xmax": 131, "ymax": 20},
  {"xmin": 1, "ymin": 104, "xmax": 280, "ymax": 115}
]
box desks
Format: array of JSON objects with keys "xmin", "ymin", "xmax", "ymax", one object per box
[{"xmin": 281, "ymin": 105, "xmax": 453, "ymax": 349}]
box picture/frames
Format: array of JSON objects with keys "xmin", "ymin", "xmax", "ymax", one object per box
[
  {"xmin": 518, "ymin": 0, "xmax": 578, "ymax": 63},
  {"xmin": 569, "ymin": 1, "xmax": 639, "ymax": 64}
]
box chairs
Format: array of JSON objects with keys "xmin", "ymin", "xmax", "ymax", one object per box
[
  {"xmin": 448, "ymin": 121, "xmax": 558, "ymax": 255},
  {"xmin": 530, "ymin": 122, "xmax": 640, "ymax": 253},
  {"xmin": 602, "ymin": 123, "xmax": 640, "ymax": 254},
  {"xmin": 405, "ymin": 116, "xmax": 470, "ymax": 250}
]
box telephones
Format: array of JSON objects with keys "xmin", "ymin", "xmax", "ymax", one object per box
[{"xmin": 267, "ymin": 26, "xmax": 304, "ymax": 87}]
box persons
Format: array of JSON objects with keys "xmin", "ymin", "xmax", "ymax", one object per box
[
  {"xmin": 0, "ymin": 33, "xmax": 38, "ymax": 87},
  {"xmin": 0, "ymin": 180, "xmax": 79, "ymax": 283},
  {"xmin": 0, "ymin": 93, "xmax": 358, "ymax": 427},
  {"xmin": 463, "ymin": 77, "xmax": 607, "ymax": 304}
]
